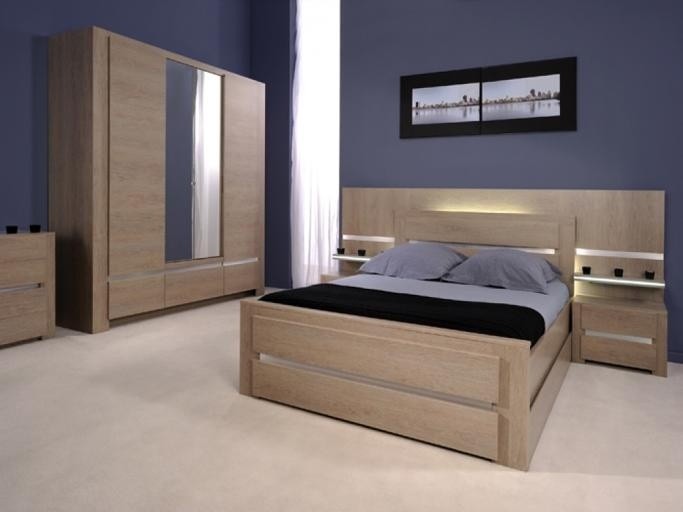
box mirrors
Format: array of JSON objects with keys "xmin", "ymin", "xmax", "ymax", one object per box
[{"xmin": 164, "ymin": 58, "xmax": 223, "ymax": 263}]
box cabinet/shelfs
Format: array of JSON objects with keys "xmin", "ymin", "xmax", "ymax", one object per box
[
  {"xmin": 48, "ymin": 26, "xmax": 266, "ymax": 335},
  {"xmin": 0, "ymin": 231, "xmax": 56, "ymax": 346}
]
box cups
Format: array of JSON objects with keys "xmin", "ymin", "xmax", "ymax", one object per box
[
  {"xmin": 338, "ymin": 247, "xmax": 345, "ymax": 254},
  {"xmin": 5, "ymin": 224, "xmax": 18, "ymax": 233},
  {"xmin": 644, "ymin": 270, "xmax": 655, "ymax": 279},
  {"xmin": 30, "ymin": 224, "xmax": 41, "ymax": 233},
  {"xmin": 615, "ymin": 268, "xmax": 623, "ymax": 277},
  {"xmin": 357, "ymin": 248, "xmax": 366, "ymax": 256},
  {"xmin": 582, "ymin": 266, "xmax": 591, "ymax": 274}
]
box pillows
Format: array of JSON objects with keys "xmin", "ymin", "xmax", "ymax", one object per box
[{"xmin": 358, "ymin": 244, "xmax": 563, "ymax": 296}]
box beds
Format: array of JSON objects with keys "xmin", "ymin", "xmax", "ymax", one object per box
[{"xmin": 239, "ymin": 208, "xmax": 578, "ymax": 473}]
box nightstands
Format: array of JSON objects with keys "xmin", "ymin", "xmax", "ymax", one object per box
[{"xmin": 571, "ymin": 295, "xmax": 668, "ymax": 378}]
